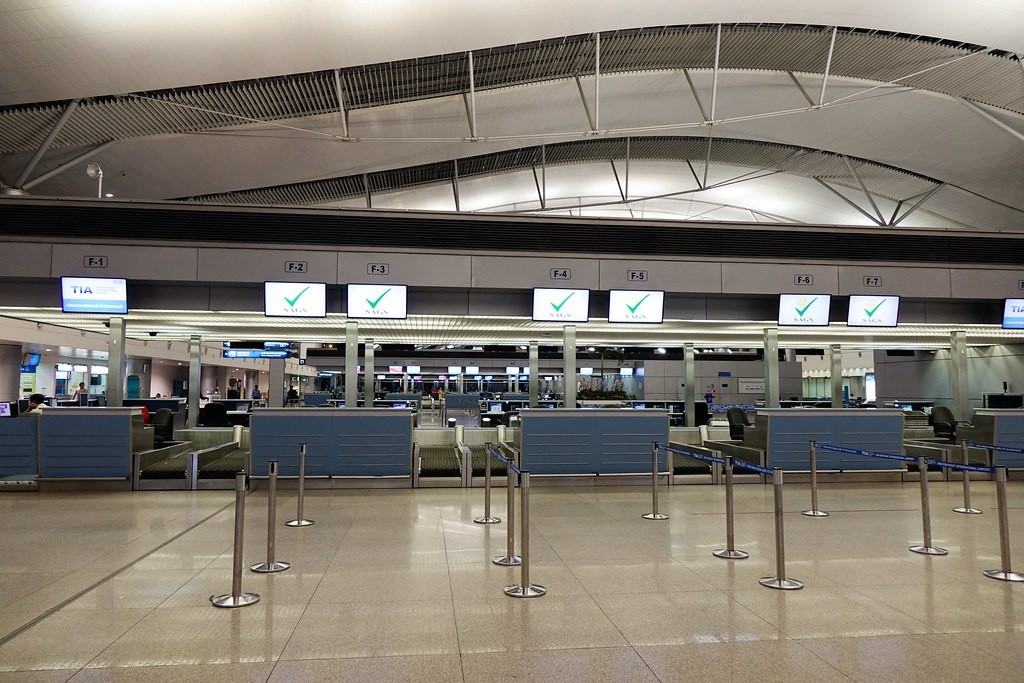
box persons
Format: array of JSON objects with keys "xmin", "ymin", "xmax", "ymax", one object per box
[
  {"xmin": 24, "ymin": 394, "xmax": 50, "ymax": 415},
  {"xmin": 287, "ymin": 386, "xmax": 297, "ymax": 405},
  {"xmin": 251, "ymin": 385, "xmax": 260, "ymax": 399},
  {"xmin": 73, "ymin": 382, "xmax": 87, "ymax": 401}
]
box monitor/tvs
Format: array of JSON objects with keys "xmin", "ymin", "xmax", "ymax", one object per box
[
  {"xmin": 633, "ymin": 402, "xmax": 645, "ymax": 408},
  {"xmin": 608, "ymin": 289, "xmax": 665, "ymax": 324},
  {"xmin": 264, "ymin": 280, "xmax": 328, "ymax": 318},
  {"xmin": 532, "ymin": 287, "xmax": 590, "ymax": 322},
  {"xmin": 580, "ymin": 368, "xmax": 593, "ymax": 375},
  {"xmin": 778, "ymin": 293, "xmax": 831, "ymax": 327},
  {"xmin": 394, "ymin": 402, "xmax": 407, "ymax": 407},
  {"xmin": 1002, "ymin": 298, "xmax": 1024, "ymax": 329},
  {"xmin": 389, "ymin": 366, "xmax": 402, "ymax": 373},
  {"xmin": 0, "ymin": 401, "xmax": 11, "ymax": 417},
  {"xmin": 407, "ymin": 366, "xmax": 420, "ymax": 374},
  {"xmin": 490, "ymin": 403, "xmax": 502, "ymax": 411},
  {"xmin": 24, "ymin": 353, "xmax": 41, "ymax": 366},
  {"xmin": 620, "ymin": 367, "xmax": 633, "ymax": 375},
  {"xmin": 466, "ymin": 367, "xmax": 479, "ymax": 374},
  {"xmin": 448, "ymin": 366, "xmax": 461, "ymax": 374},
  {"xmin": 847, "ymin": 294, "xmax": 900, "ymax": 327},
  {"xmin": 524, "ymin": 367, "xmax": 529, "ymax": 374},
  {"xmin": 60, "ymin": 276, "xmax": 128, "ymax": 315},
  {"xmin": 506, "ymin": 367, "xmax": 519, "ymax": 374},
  {"xmin": 346, "ymin": 283, "xmax": 408, "ymax": 319}
]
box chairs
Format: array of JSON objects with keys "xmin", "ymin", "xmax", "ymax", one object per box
[
  {"xmin": 683, "ymin": 401, "xmax": 714, "ymax": 427},
  {"xmin": 204, "ymin": 402, "xmax": 228, "ymax": 427},
  {"xmin": 931, "ymin": 406, "xmax": 973, "ymax": 446},
  {"xmin": 144, "ymin": 409, "xmax": 172, "ymax": 443},
  {"xmin": 726, "ymin": 407, "xmax": 753, "ymax": 440},
  {"xmin": 496, "ymin": 411, "xmax": 519, "ymax": 427}
]
